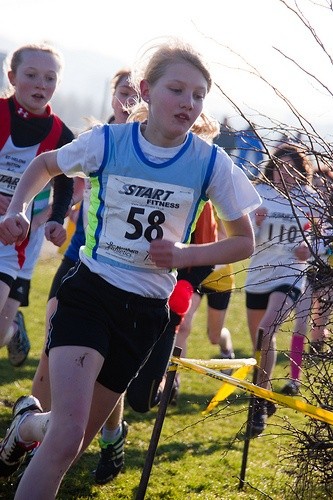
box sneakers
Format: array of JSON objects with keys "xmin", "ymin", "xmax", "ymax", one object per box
[
  {"xmin": 220, "ymin": 347, "xmax": 235, "ymax": 376},
  {"xmin": 7, "ymin": 310, "xmax": 31, "ymax": 366},
  {"xmin": 250, "ymin": 397, "xmax": 267, "ymax": 439},
  {"xmin": 265, "ymin": 401, "xmax": 276, "ymax": 418},
  {"xmin": 95, "ymin": 420, "xmax": 129, "ymax": 485},
  {"xmin": 279, "ymin": 379, "xmax": 301, "ymax": 396},
  {"xmin": 0, "ymin": 394, "xmax": 43, "ymax": 478}
]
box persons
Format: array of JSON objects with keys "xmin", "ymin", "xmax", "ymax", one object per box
[
  {"xmin": 0, "ymin": 48, "xmax": 333, "ymax": 485},
  {"xmin": 0, "ymin": 45, "xmax": 262, "ymax": 500}
]
